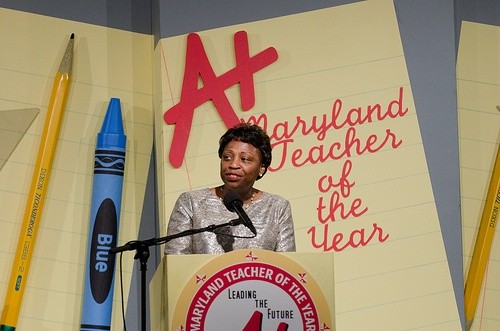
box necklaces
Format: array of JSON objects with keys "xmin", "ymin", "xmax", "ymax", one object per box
[{"xmin": 219, "ymin": 185, "xmax": 256, "ymax": 205}]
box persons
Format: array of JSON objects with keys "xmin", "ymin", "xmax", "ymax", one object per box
[{"xmin": 162, "ymin": 124, "xmax": 295, "ymax": 254}]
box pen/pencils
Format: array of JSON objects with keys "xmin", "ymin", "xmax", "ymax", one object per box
[
  {"xmin": 462, "ymin": 144, "xmax": 500, "ymax": 331},
  {"xmin": 76, "ymin": 98, "xmax": 129, "ymax": 331},
  {"xmin": 0, "ymin": 31, "xmax": 78, "ymax": 331}
]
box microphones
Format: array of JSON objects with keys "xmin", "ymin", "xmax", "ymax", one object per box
[{"xmin": 222, "ymin": 190, "xmax": 256, "ymax": 233}]
